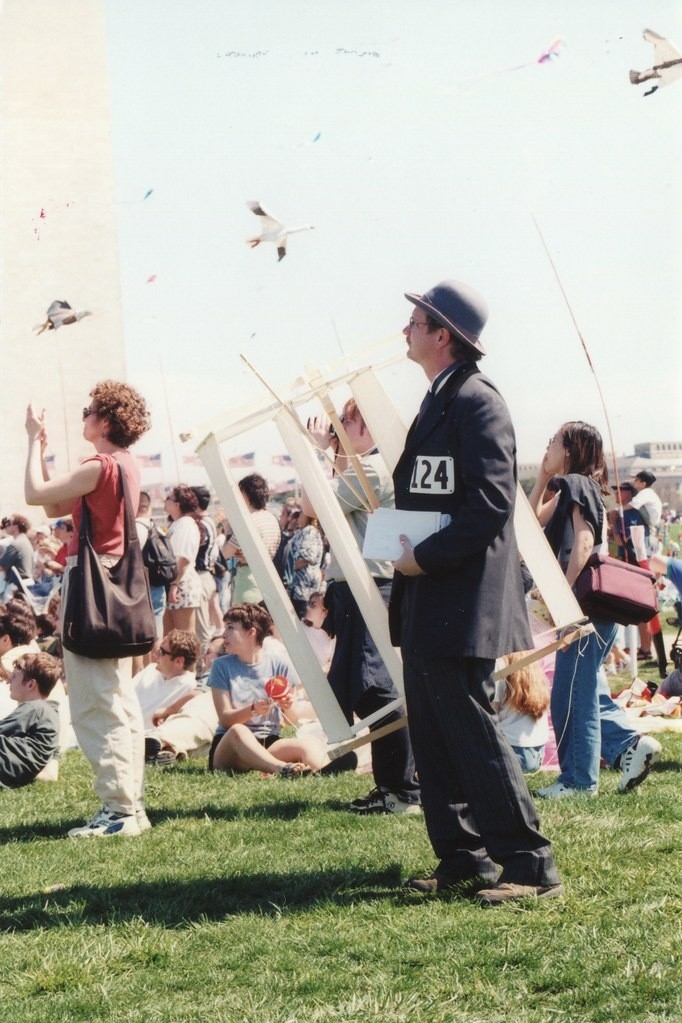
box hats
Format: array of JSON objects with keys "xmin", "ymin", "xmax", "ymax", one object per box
[
  {"xmin": 404, "ymin": 279, "xmax": 491, "ymax": 356},
  {"xmin": 36, "ymin": 526, "xmax": 51, "ymax": 537},
  {"xmin": 611, "ymin": 482, "xmax": 638, "ymax": 496}
]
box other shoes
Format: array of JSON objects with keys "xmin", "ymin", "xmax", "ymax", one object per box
[
  {"xmin": 313, "ymin": 751, "xmax": 358, "ymax": 778},
  {"xmin": 475, "ymin": 881, "xmax": 562, "ymax": 909},
  {"xmin": 157, "ymin": 750, "xmax": 176, "ymax": 766},
  {"xmin": 280, "ymin": 762, "xmax": 311, "ymax": 779},
  {"xmin": 145, "ymin": 735, "xmax": 162, "ymax": 764},
  {"xmin": 402, "ymin": 878, "xmax": 437, "ymax": 894},
  {"xmin": 637, "ymin": 648, "xmax": 653, "ymax": 660}
]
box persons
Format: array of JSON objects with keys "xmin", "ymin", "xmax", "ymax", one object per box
[
  {"xmin": 0, "ymin": 653, "xmax": 61, "ymax": 786},
  {"xmin": 605, "ymin": 469, "xmax": 682, "ymax": 698},
  {"xmin": 530, "ymin": 421, "xmax": 661, "ymax": 799},
  {"xmin": 24, "ymin": 380, "xmax": 153, "ymax": 837},
  {"xmin": 1, "ymin": 515, "xmax": 199, "ymax": 779},
  {"xmin": 307, "ymin": 396, "xmax": 420, "ymax": 817},
  {"xmin": 206, "ymin": 603, "xmax": 358, "ymax": 777},
  {"xmin": 388, "ymin": 283, "xmax": 563, "ymax": 905},
  {"xmin": 134, "ymin": 483, "xmax": 337, "ymax": 762}
]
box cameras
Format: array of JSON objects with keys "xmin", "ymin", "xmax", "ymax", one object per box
[{"xmin": 307, "ymin": 415, "xmax": 344, "ymax": 437}]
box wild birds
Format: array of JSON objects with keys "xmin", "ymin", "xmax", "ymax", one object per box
[
  {"xmin": 30, "ymin": 299, "xmax": 92, "ymax": 336},
  {"xmin": 629, "ymin": 28, "xmax": 682, "ymax": 97},
  {"xmin": 246, "ymin": 200, "xmax": 315, "ymax": 262}
]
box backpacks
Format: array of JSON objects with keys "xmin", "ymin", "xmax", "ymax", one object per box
[{"xmin": 135, "ymin": 518, "xmax": 178, "ymax": 587}]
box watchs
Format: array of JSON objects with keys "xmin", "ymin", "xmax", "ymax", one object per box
[{"xmin": 251, "ymin": 703, "xmax": 258, "ymax": 717}]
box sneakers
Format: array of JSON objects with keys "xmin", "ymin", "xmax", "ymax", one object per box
[
  {"xmin": 531, "ymin": 782, "xmax": 597, "ymax": 799},
  {"xmin": 67, "ymin": 805, "xmax": 152, "ymax": 840},
  {"xmin": 618, "ymin": 733, "xmax": 662, "ymax": 794},
  {"xmin": 347, "ymin": 786, "xmax": 421, "ymax": 814}
]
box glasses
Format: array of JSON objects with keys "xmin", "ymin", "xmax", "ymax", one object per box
[
  {"xmin": 160, "ymin": 647, "xmax": 172, "ymax": 657},
  {"xmin": 549, "ymin": 438, "xmax": 565, "ymax": 445},
  {"xmin": 82, "ymin": 407, "xmax": 99, "ymax": 419},
  {"xmin": 5, "ymin": 520, "xmax": 12, "ymax": 526},
  {"xmin": 409, "ymin": 316, "xmax": 438, "ymax": 331},
  {"xmin": 167, "ymin": 496, "xmax": 177, "ymax": 502}
]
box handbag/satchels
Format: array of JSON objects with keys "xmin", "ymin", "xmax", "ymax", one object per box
[
  {"xmin": 576, "ymin": 553, "xmax": 659, "ymax": 626},
  {"xmin": 61, "ymin": 456, "xmax": 158, "ymax": 658}
]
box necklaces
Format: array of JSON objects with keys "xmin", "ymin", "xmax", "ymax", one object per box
[{"xmin": 497, "ymin": 563, "xmax": 560, "ymax": 771}]
